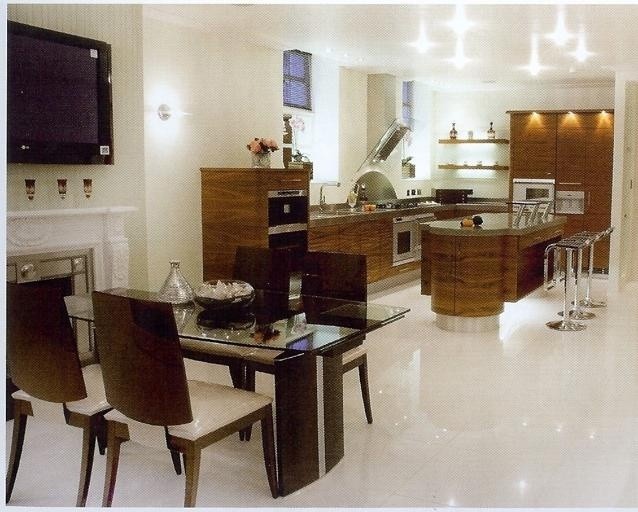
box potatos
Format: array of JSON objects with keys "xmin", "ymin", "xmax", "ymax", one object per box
[{"xmin": 464, "ymin": 219, "xmax": 473, "ymax": 227}]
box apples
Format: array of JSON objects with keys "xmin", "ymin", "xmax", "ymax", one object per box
[
  {"xmin": 370, "ymin": 205, "xmax": 376, "ymax": 211},
  {"xmin": 364, "ymin": 205, "xmax": 370, "ymax": 211}
]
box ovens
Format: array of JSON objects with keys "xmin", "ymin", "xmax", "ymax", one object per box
[{"xmin": 393, "ymin": 216, "xmax": 437, "ymax": 261}]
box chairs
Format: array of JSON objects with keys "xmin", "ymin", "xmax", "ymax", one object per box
[
  {"xmin": 231, "ymin": 246, "xmax": 292, "ymax": 441},
  {"xmin": 244, "ymin": 250, "xmax": 371, "ymax": 444},
  {"xmin": 7, "ymin": 280, "xmax": 181, "ymax": 507},
  {"xmin": 91, "ymin": 288, "xmax": 281, "ymax": 507}
]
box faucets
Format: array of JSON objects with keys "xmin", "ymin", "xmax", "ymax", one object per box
[{"xmin": 319, "ymin": 181, "xmax": 340, "ymax": 213}]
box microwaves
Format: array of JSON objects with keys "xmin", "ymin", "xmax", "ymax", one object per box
[{"xmin": 513, "ymin": 179, "xmax": 555, "ymax": 213}]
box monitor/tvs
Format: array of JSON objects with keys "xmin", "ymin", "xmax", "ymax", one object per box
[{"xmin": 8, "ymin": 19, "xmax": 113, "ymax": 165}]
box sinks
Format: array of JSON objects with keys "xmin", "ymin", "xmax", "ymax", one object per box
[{"xmin": 323, "ymin": 211, "xmax": 366, "ymax": 215}]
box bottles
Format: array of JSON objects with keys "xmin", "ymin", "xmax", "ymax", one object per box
[
  {"xmin": 360, "ymin": 184, "xmax": 368, "ymax": 208},
  {"xmin": 450, "ymin": 123, "xmax": 457, "ymax": 139},
  {"xmin": 487, "ymin": 121, "xmax": 495, "ymax": 139}
]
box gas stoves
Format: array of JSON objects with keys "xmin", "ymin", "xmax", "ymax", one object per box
[{"xmin": 375, "ymin": 202, "xmax": 418, "ymax": 210}]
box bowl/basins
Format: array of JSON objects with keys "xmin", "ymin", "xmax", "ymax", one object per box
[{"xmin": 194, "ymin": 279, "xmax": 257, "ymax": 311}]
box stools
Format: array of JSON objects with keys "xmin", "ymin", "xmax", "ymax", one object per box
[{"xmin": 540, "ymin": 225, "xmax": 617, "ymax": 333}]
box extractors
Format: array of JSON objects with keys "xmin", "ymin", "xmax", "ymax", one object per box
[{"xmin": 366, "ymin": 73, "xmax": 408, "ymax": 165}]
